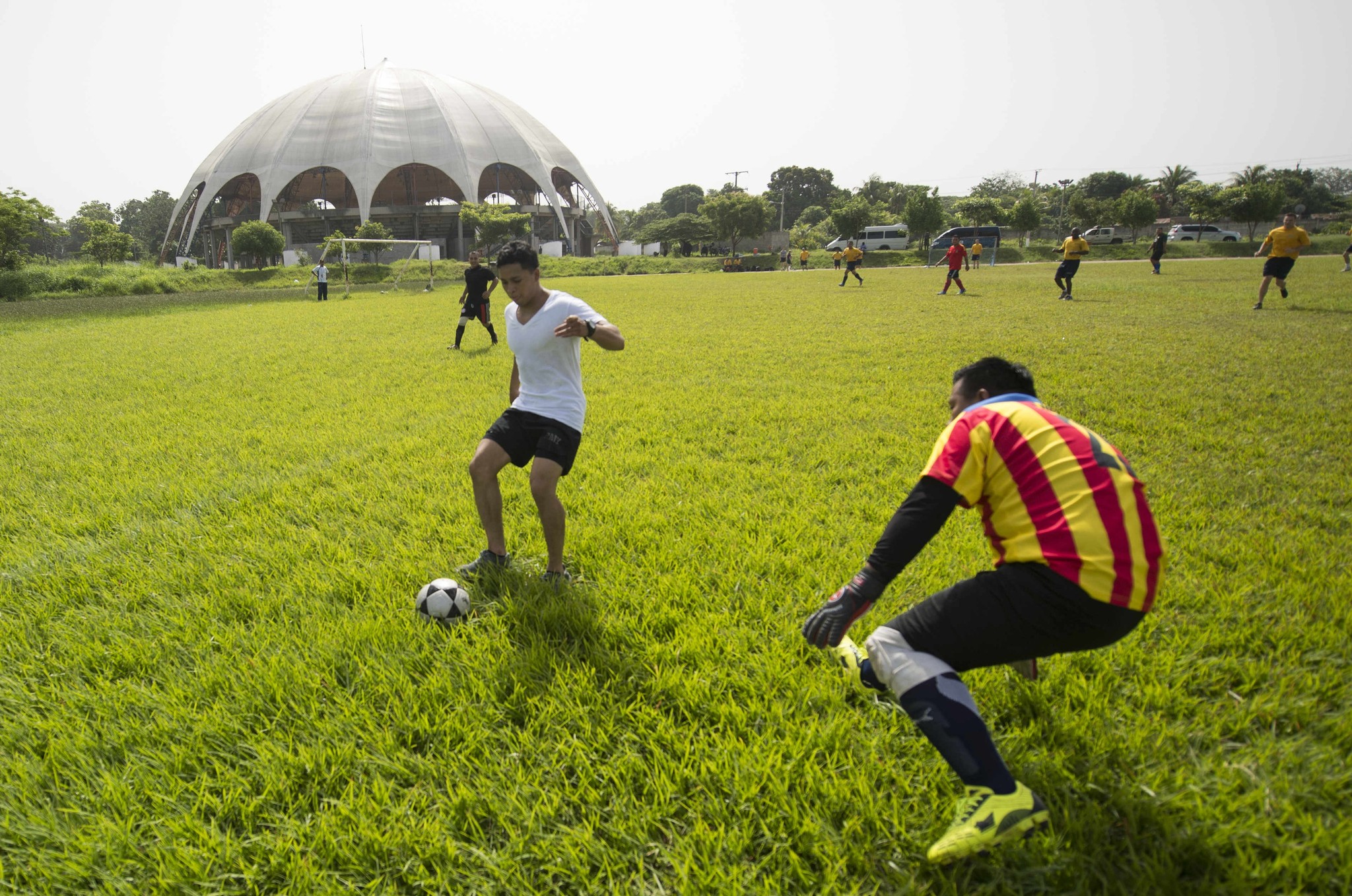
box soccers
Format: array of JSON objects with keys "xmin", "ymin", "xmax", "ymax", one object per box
[{"xmin": 416, "ymin": 578, "xmax": 471, "ymax": 624}]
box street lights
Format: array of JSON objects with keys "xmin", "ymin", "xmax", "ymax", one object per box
[
  {"xmin": 769, "ymin": 188, "xmax": 785, "ymax": 232},
  {"xmin": 271, "ymin": 198, "xmax": 285, "ymax": 233},
  {"xmin": 1057, "ymin": 179, "xmax": 1074, "ymax": 246},
  {"xmin": 509, "ymin": 189, "xmax": 523, "ymax": 213}
]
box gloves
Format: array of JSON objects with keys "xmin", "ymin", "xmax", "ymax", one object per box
[{"xmin": 801, "ymin": 574, "xmax": 881, "ymax": 649}]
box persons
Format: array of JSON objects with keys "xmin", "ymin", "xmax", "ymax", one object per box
[
  {"xmin": 1052, "ymin": 227, "xmax": 1089, "ymax": 300},
  {"xmin": 1252, "ymin": 213, "xmax": 1311, "ymax": 309},
  {"xmin": 935, "ymin": 235, "xmax": 970, "ymax": 295},
  {"xmin": 1146, "ymin": 227, "xmax": 1168, "ymax": 275},
  {"xmin": 1340, "ymin": 226, "xmax": 1352, "ymax": 272},
  {"xmin": 923, "ymin": 231, "xmax": 931, "ymax": 250},
  {"xmin": 312, "ymin": 259, "xmax": 330, "ymax": 301},
  {"xmin": 837, "ymin": 241, "xmax": 864, "ymax": 286},
  {"xmin": 968, "ymin": 239, "xmax": 982, "ymax": 269},
  {"xmin": 662, "ymin": 241, "xmax": 866, "ymax": 272},
  {"xmin": 447, "ymin": 251, "xmax": 499, "ymax": 350},
  {"xmin": 803, "ymin": 358, "xmax": 1164, "ymax": 861},
  {"xmin": 452, "ymin": 240, "xmax": 625, "ymax": 583},
  {"xmin": 538, "ymin": 246, "xmax": 542, "ymax": 254}
]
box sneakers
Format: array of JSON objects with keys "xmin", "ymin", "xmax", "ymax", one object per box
[
  {"xmin": 926, "ymin": 779, "xmax": 1050, "ymax": 865},
  {"xmin": 1063, "ymin": 295, "xmax": 1072, "ymax": 300},
  {"xmin": 460, "ymin": 550, "xmax": 509, "ymax": 576},
  {"xmin": 447, "ymin": 345, "xmax": 460, "ymax": 350},
  {"xmin": 827, "ymin": 633, "xmax": 888, "ymax": 702},
  {"xmin": 1058, "ymin": 290, "xmax": 1067, "ymax": 299},
  {"xmin": 491, "ymin": 333, "xmax": 498, "ymax": 345},
  {"xmin": 540, "ymin": 564, "xmax": 571, "ymax": 591}
]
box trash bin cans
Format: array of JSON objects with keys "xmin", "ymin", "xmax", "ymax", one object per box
[
  {"xmin": 753, "ymin": 248, "xmax": 758, "ymax": 254},
  {"xmin": 489, "ymin": 262, "xmax": 500, "ymax": 279}
]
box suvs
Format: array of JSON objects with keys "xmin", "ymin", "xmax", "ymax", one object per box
[
  {"xmin": 594, "ymin": 240, "xmax": 628, "ymax": 257},
  {"xmin": 1166, "ymin": 225, "xmax": 1242, "ymax": 244}
]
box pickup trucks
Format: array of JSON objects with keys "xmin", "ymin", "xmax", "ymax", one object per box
[{"xmin": 1065, "ymin": 224, "xmax": 1133, "ymax": 245}]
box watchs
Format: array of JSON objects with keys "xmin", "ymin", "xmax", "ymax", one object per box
[{"xmin": 582, "ymin": 318, "xmax": 596, "ymax": 342}]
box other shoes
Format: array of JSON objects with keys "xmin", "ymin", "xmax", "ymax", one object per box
[
  {"xmin": 1253, "ymin": 303, "xmax": 1263, "ymax": 309},
  {"xmin": 1279, "ymin": 286, "xmax": 1288, "ymax": 298},
  {"xmin": 937, "ymin": 291, "xmax": 946, "ymax": 295},
  {"xmin": 1340, "ymin": 267, "xmax": 1351, "ymax": 272},
  {"xmin": 1150, "ymin": 269, "xmax": 1160, "ymax": 274},
  {"xmin": 957, "ymin": 289, "xmax": 966, "ymax": 295},
  {"xmin": 859, "ymin": 278, "xmax": 863, "ymax": 285},
  {"xmin": 839, "ymin": 283, "xmax": 844, "ymax": 286}
]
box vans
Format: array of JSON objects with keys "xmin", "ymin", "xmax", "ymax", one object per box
[
  {"xmin": 824, "ymin": 223, "xmax": 910, "ymax": 252},
  {"xmin": 930, "ymin": 225, "xmax": 1001, "ymax": 250}
]
